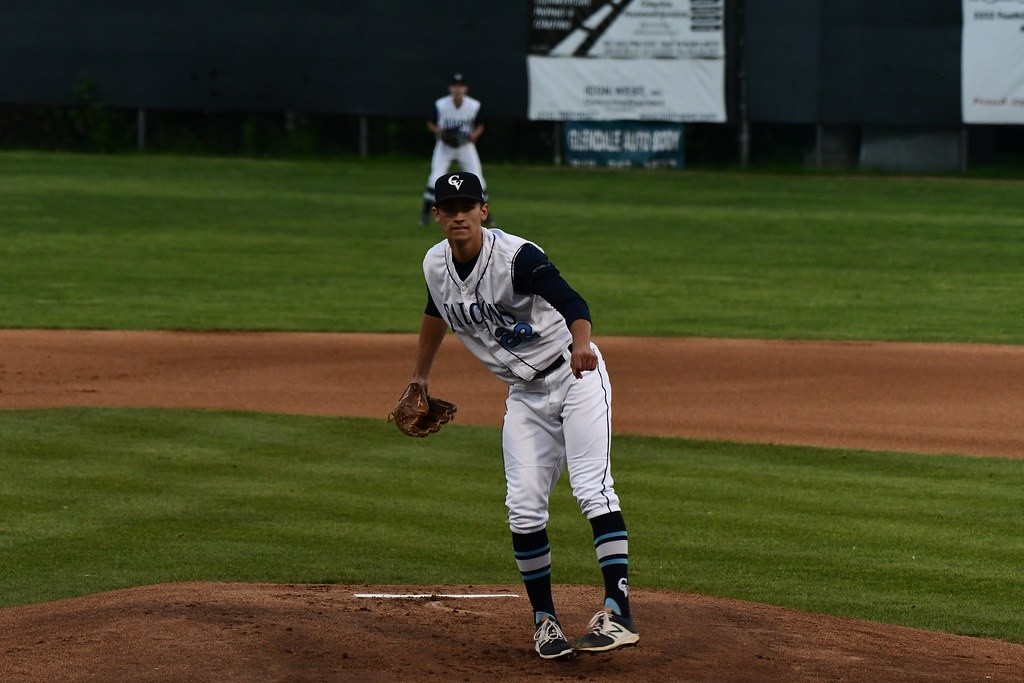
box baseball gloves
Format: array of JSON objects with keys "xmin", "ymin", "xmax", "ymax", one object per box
[{"xmin": 387, "ymin": 383, "xmax": 459, "ymax": 437}]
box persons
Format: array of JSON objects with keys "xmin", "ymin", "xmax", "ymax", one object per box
[
  {"xmin": 390, "ymin": 173, "xmax": 640, "ymax": 660},
  {"xmin": 417, "ymin": 73, "xmax": 494, "ymax": 228}
]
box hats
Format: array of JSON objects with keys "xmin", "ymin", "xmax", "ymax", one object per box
[
  {"xmin": 434, "ymin": 172, "xmax": 484, "ymax": 209},
  {"xmin": 448, "ymin": 73, "xmax": 468, "ymax": 85}
]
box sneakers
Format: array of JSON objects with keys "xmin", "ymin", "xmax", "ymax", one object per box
[
  {"xmin": 534, "ymin": 616, "xmax": 574, "ymax": 658},
  {"xmin": 574, "ymin": 610, "xmax": 640, "ymax": 651}
]
